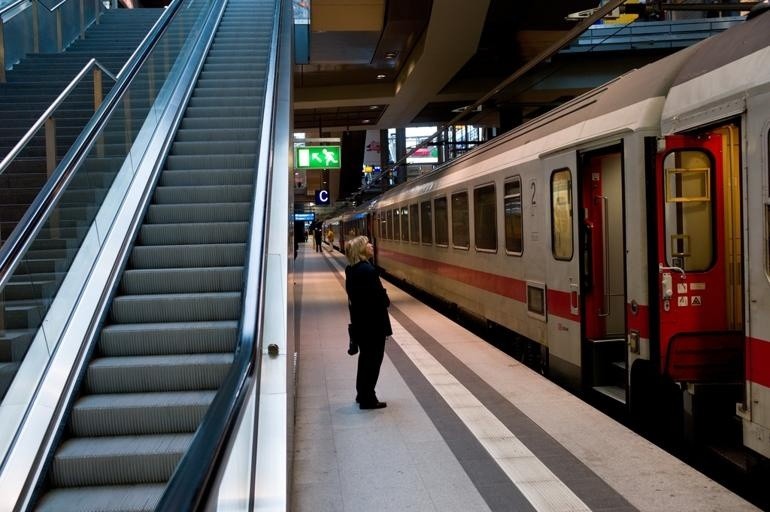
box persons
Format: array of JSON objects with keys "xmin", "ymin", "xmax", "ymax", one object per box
[
  {"xmin": 343, "ymin": 233, "xmax": 394, "ymax": 411},
  {"xmin": 303, "ymin": 225, "xmax": 335, "ymax": 254}
]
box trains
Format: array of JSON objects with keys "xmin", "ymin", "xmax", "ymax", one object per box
[{"xmin": 321, "ymin": 1, "xmax": 770, "ymax": 506}]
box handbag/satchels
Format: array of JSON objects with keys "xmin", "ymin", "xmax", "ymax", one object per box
[{"xmin": 348, "ymin": 324, "xmax": 358, "ymax": 356}]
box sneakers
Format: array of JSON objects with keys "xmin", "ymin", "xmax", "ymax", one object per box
[{"xmin": 360, "ymin": 402, "xmax": 386, "ymax": 408}]
